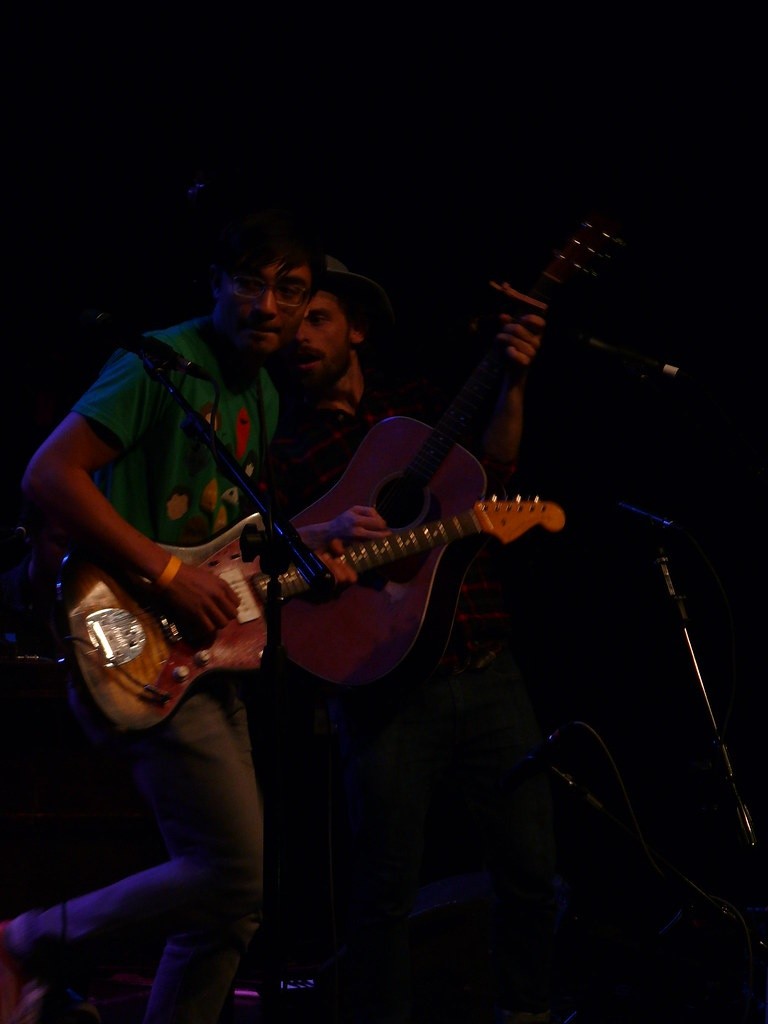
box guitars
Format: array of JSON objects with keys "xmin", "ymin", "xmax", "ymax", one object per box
[
  {"xmin": 64, "ymin": 493, "xmax": 565, "ymax": 730},
  {"xmin": 259, "ymin": 219, "xmax": 623, "ymax": 690}
]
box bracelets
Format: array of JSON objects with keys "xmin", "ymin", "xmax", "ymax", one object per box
[{"xmin": 143, "ymin": 549, "xmax": 182, "ymax": 593}]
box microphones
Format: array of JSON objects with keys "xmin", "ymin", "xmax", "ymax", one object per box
[
  {"xmin": 94, "ymin": 312, "xmax": 212, "ymax": 382},
  {"xmin": 14, "ymin": 512, "xmax": 31, "ymax": 539},
  {"xmin": 500, "ymin": 722, "xmax": 578, "ymax": 781},
  {"xmin": 617, "ymin": 503, "xmax": 679, "ymax": 529},
  {"xmin": 589, "ymin": 336, "xmax": 680, "ymax": 378}
]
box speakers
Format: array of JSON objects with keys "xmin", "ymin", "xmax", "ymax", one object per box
[{"xmin": 290, "ymin": 637, "xmax": 560, "ymax": 1024}]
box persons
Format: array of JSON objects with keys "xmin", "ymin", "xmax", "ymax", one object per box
[{"xmin": 0, "ymin": 209, "xmax": 551, "ymax": 1024}]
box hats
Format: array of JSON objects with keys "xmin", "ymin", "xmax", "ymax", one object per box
[{"xmin": 318, "ymin": 252, "xmax": 395, "ymax": 341}]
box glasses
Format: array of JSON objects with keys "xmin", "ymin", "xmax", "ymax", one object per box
[{"xmin": 221, "ymin": 260, "xmax": 313, "ymax": 310}]
